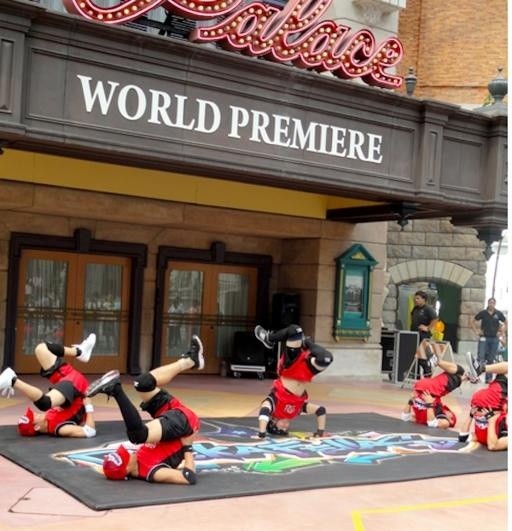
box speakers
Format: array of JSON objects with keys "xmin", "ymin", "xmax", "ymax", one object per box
[
  {"xmin": 233, "ymin": 331, "xmax": 267, "ymax": 378},
  {"xmin": 380, "ymin": 327, "xmax": 395, "ymax": 373},
  {"xmin": 393, "ymin": 331, "xmax": 420, "ymax": 384},
  {"xmin": 272, "ymin": 293, "xmax": 300, "ymax": 370}
]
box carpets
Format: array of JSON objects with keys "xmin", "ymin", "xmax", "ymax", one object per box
[{"xmin": 0, "ymin": 412, "xmax": 508, "ymax": 511}]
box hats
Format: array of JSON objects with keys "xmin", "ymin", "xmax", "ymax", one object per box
[
  {"xmin": 266, "ymin": 420, "xmax": 288, "ymax": 436},
  {"xmin": 443, "ymin": 404, "xmax": 456, "ymax": 426},
  {"xmin": 103, "ymin": 446, "xmax": 130, "ymax": 479},
  {"xmin": 18, "ymin": 409, "xmax": 39, "ymax": 437}
]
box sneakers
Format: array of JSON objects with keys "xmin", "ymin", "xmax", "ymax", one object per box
[
  {"xmin": 425, "ymin": 346, "xmax": 436, "ymax": 368},
  {"xmin": 187, "ymin": 336, "xmax": 205, "ymax": 372},
  {"xmin": 0, "ymin": 367, "xmax": 17, "ymax": 393},
  {"xmin": 86, "ymin": 369, "xmax": 121, "ymax": 398},
  {"xmin": 76, "ymin": 333, "xmax": 96, "ymax": 364},
  {"xmin": 467, "ymin": 352, "xmax": 487, "ymax": 378},
  {"xmin": 255, "ymin": 325, "xmax": 276, "ymax": 349}
]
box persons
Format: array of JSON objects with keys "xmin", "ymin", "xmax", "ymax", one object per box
[
  {"xmin": 403, "ymin": 340, "xmax": 465, "ymax": 428},
  {"xmin": 409, "ymin": 290, "xmax": 439, "ymax": 378},
  {"xmin": 21, "ymin": 286, "xmax": 221, "ymax": 357},
  {"xmin": 253, "ymin": 321, "xmax": 334, "ymax": 444},
  {"xmin": 458, "ymin": 345, "xmax": 509, "ymax": 452},
  {"xmin": 86, "ymin": 333, "xmax": 205, "ymax": 486},
  {"xmin": 472, "ymin": 298, "xmax": 507, "ymax": 382},
  {"xmin": 1, "ymin": 332, "xmax": 97, "ymax": 438}
]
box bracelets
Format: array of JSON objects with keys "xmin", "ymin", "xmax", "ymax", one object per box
[
  {"xmin": 83, "ymin": 404, "xmax": 94, "ymax": 413},
  {"xmin": 483, "ymin": 412, "xmax": 495, "ymax": 420},
  {"xmin": 317, "ymin": 427, "xmax": 326, "ymax": 436},
  {"xmin": 180, "ymin": 443, "xmax": 193, "ymax": 452},
  {"xmin": 424, "ymin": 401, "xmax": 433, "ymax": 408},
  {"xmin": 257, "ymin": 429, "xmax": 267, "ymax": 439}
]
